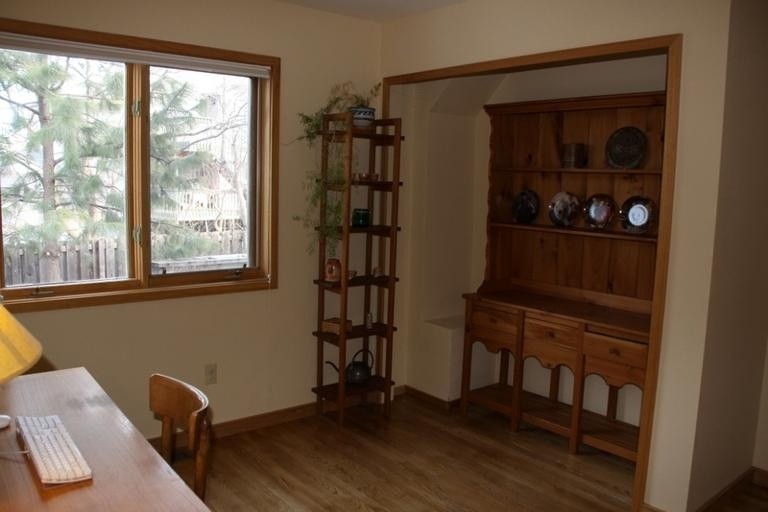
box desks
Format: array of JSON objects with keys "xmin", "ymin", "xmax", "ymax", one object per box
[{"xmin": 0, "ymin": 366, "xmax": 212, "ymax": 512}]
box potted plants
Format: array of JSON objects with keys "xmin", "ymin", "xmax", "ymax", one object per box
[{"xmin": 296, "ymin": 82, "xmax": 376, "ymax": 255}]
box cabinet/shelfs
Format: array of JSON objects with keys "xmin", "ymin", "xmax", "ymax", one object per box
[
  {"xmin": 310, "ymin": 112, "xmax": 406, "ymax": 426},
  {"xmin": 483, "ymin": 89, "xmax": 666, "ymax": 299},
  {"xmin": 459, "ymin": 287, "xmax": 652, "ymax": 464}
]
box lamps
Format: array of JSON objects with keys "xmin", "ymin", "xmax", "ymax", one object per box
[{"xmin": 0, "ymin": 301, "xmax": 43, "ymax": 386}]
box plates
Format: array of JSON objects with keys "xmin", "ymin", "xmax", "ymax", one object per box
[
  {"xmin": 604, "ymin": 124, "xmax": 652, "ymax": 171},
  {"xmin": 510, "ymin": 190, "xmax": 656, "ymax": 236}
]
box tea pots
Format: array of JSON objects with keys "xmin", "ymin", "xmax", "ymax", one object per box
[{"xmin": 322, "ymin": 347, "xmax": 378, "ymax": 392}]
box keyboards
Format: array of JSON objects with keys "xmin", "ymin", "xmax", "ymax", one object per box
[{"xmin": 15, "ymin": 415, "xmax": 93, "ymax": 485}]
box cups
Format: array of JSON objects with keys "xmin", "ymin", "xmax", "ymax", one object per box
[
  {"xmin": 352, "ymin": 208, "xmax": 370, "ymax": 229},
  {"xmin": 359, "ymin": 172, "xmax": 379, "ymax": 182}
]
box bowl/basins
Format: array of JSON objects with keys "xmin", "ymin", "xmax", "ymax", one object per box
[{"xmin": 557, "ymin": 143, "xmax": 594, "ymax": 168}]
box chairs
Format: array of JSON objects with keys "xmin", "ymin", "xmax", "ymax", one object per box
[{"xmin": 149, "ymin": 373, "xmax": 212, "ymax": 501}]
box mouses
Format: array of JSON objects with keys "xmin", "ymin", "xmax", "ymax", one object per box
[{"xmin": 0, "ymin": 415, "xmax": 11, "ymax": 429}]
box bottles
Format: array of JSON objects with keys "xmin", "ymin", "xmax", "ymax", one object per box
[{"xmin": 323, "ymin": 258, "xmax": 342, "ymax": 284}]
